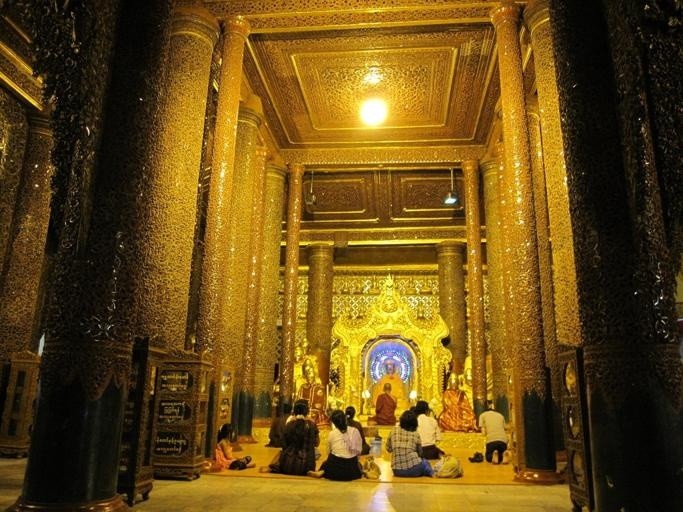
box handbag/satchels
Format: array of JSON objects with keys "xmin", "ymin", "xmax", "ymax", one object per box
[{"xmin": 434, "ymin": 453, "xmax": 464, "ymax": 478}]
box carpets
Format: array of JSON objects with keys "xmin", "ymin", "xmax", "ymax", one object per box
[{"xmin": 202, "ymin": 426, "xmax": 518, "ymax": 484}]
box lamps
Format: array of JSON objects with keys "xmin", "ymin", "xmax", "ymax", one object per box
[{"xmin": 444, "ymin": 166, "xmax": 459, "ymax": 205}]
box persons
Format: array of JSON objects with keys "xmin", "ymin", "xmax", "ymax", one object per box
[
  {"xmin": 478, "ymin": 407, "xmax": 511, "ymax": 465},
  {"xmin": 367, "ymin": 382, "xmax": 396, "ymax": 426},
  {"xmin": 437, "ymin": 377, "xmax": 480, "ymax": 433},
  {"xmin": 259, "ymin": 400, "xmax": 447, "ymax": 481},
  {"xmin": 370, "ymin": 361, "xmax": 411, "ymax": 408},
  {"xmin": 290, "ymin": 362, "xmax": 332, "ymax": 429},
  {"xmin": 214, "ymin": 423, "xmax": 256, "ymax": 470}
]
